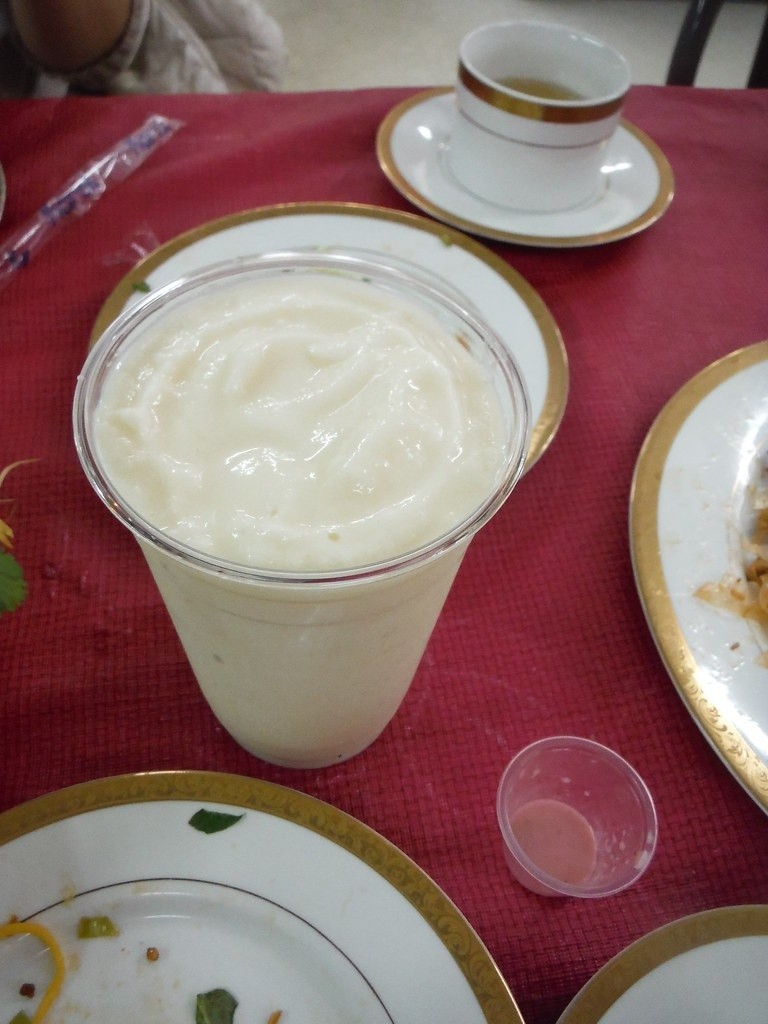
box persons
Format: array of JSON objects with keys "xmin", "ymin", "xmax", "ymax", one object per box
[{"xmin": 0, "ymin": 0, "xmax": 290, "ymax": 98}]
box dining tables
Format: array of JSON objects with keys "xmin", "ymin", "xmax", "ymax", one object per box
[{"xmin": 0, "ymin": 84, "xmax": 768, "ymax": 1024}]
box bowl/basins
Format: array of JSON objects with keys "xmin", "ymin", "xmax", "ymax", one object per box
[{"xmin": 496, "ymin": 737, "xmax": 658, "ymax": 899}]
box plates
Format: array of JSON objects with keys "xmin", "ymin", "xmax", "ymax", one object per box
[
  {"xmin": 90, "ymin": 202, "xmax": 568, "ymax": 478},
  {"xmin": 375, "ymin": 86, "xmax": 675, "ymax": 248},
  {"xmin": 556, "ymin": 905, "xmax": 767, "ymax": 1023},
  {"xmin": 630, "ymin": 340, "xmax": 768, "ymax": 816},
  {"xmin": 1, "ymin": 769, "xmax": 525, "ymax": 1023}
]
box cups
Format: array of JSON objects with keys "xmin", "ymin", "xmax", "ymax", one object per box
[
  {"xmin": 73, "ymin": 251, "xmax": 530, "ymax": 768},
  {"xmin": 451, "ymin": 23, "xmax": 631, "ymax": 212}
]
